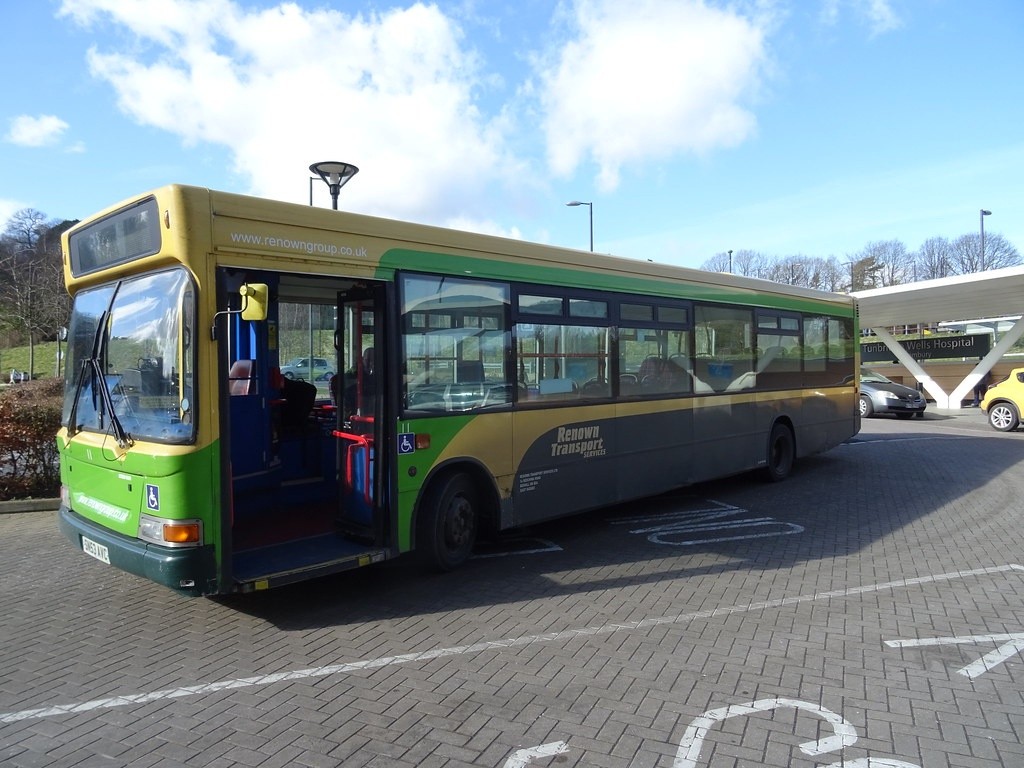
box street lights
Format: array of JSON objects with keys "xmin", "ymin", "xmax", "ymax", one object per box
[
  {"xmin": 566, "ymin": 201, "xmax": 594, "ymax": 252},
  {"xmin": 310, "ymin": 162, "xmax": 360, "ymax": 370},
  {"xmin": 980, "ymin": 209, "xmax": 992, "ymax": 274}
]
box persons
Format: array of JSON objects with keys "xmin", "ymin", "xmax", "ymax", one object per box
[
  {"xmin": 971, "ymin": 354, "xmax": 990, "ymax": 411},
  {"xmin": 346, "ymin": 344, "xmax": 378, "ymax": 423}
]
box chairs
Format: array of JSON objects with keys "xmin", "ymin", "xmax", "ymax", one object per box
[{"xmin": 315, "ymin": 339, "xmax": 843, "ymax": 438}]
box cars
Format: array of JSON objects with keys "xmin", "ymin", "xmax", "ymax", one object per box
[
  {"xmin": 979, "ymin": 368, "xmax": 1023, "ymax": 432},
  {"xmin": 279, "ymin": 358, "xmax": 337, "ymax": 382},
  {"xmin": 859, "ymin": 365, "xmax": 927, "ymax": 421}
]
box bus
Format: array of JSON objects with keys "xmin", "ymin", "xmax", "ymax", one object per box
[{"xmin": 55, "ymin": 183, "xmax": 862, "ymax": 600}]
